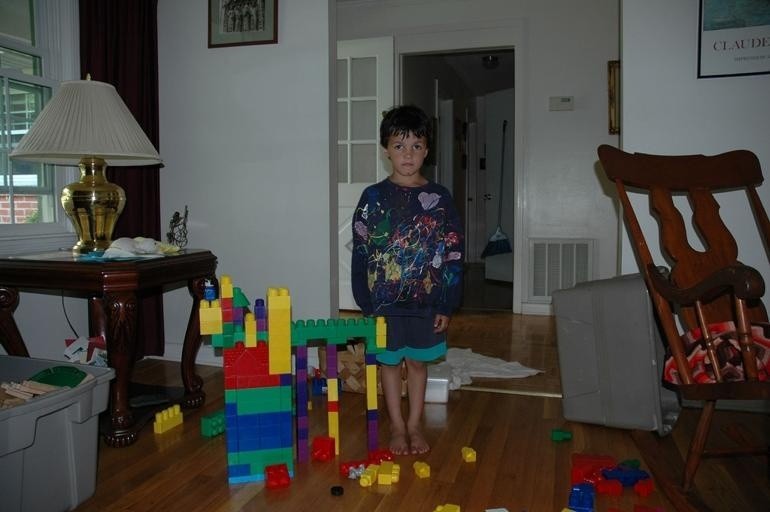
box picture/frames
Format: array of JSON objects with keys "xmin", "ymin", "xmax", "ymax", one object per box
[
  {"xmin": 208, "ymin": 0, "xmax": 279, "ymax": 48},
  {"xmin": 696, "ymin": 0, "xmax": 770, "ymax": 79}
]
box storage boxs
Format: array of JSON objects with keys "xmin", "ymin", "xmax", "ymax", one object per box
[{"xmin": 0, "ymin": 353, "xmax": 116, "ymax": 511}]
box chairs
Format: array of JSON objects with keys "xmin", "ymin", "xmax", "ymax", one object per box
[{"xmin": 597, "ymin": 138, "xmax": 770, "ymax": 490}]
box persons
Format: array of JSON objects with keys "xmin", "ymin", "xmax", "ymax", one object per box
[
  {"xmin": 221, "ymin": 0, "xmax": 259, "ymax": 33},
  {"xmin": 351, "ymin": 103, "xmax": 465, "ymax": 454}
]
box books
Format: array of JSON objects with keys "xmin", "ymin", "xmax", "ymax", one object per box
[
  {"xmin": 1, "ymin": 378, "xmax": 71, "ymax": 412},
  {"xmin": 63, "ymin": 336, "xmax": 110, "ymax": 367}
]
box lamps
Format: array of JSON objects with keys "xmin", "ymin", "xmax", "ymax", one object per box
[{"xmin": 10, "ymin": 73, "xmax": 162, "ymax": 255}]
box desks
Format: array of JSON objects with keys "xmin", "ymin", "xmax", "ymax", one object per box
[{"xmin": 0, "ymin": 250, "xmax": 221, "ymax": 448}]
